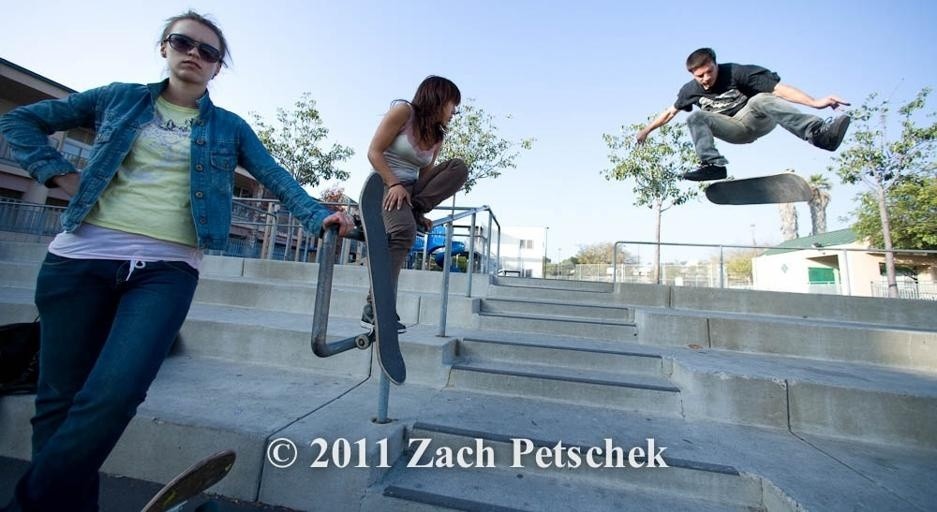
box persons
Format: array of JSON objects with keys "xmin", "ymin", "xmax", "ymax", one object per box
[
  {"xmin": 636, "ymin": 47, "xmax": 848, "ymax": 181},
  {"xmin": 0, "ymin": 8, "xmax": 355, "ymax": 511},
  {"xmin": 360, "ymin": 73, "xmax": 468, "ymax": 334}
]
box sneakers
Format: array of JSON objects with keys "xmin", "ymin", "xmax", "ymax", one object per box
[
  {"xmin": 812, "ymin": 114, "xmax": 850, "ymax": 151},
  {"xmin": 684, "ymin": 165, "xmax": 727, "ymax": 180},
  {"xmin": 360, "ymin": 305, "xmax": 406, "ymax": 334}
]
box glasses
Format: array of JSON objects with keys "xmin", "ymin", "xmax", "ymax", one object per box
[{"xmin": 163, "ymin": 33, "xmax": 222, "ymax": 63}]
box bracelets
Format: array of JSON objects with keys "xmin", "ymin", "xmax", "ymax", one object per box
[{"xmin": 388, "ymin": 183, "xmax": 404, "ymax": 190}]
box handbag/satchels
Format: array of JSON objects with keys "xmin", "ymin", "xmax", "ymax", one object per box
[{"xmin": 1, "ymin": 321, "xmax": 42, "ymax": 395}]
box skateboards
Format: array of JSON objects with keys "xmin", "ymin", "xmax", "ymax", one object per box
[
  {"xmin": 141, "ymin": 449, "xmax": 235, "ymax": 512},
  {"xmin": 354, "ymin": 172, "xmax": 405, "ymax": 385},
  {"xmin": 706, "ymin": 169, "xmax": 814, "ymax": 205}
]
